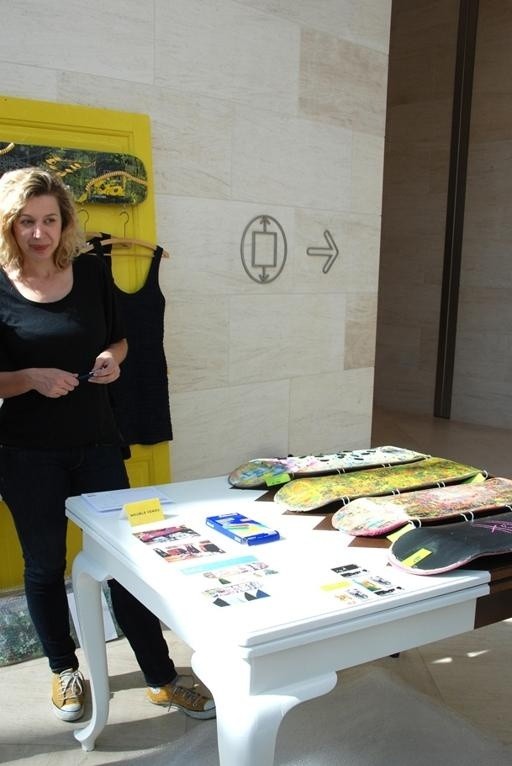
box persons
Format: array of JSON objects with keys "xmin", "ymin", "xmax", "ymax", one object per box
[{"xmin": 0, "ymin": 166, "xmax": 215, "ymax": 721}]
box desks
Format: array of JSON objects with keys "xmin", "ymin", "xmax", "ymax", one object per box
[{"xmin": 61, "ymin": 446, "xmax": 512, "ymax": 766}]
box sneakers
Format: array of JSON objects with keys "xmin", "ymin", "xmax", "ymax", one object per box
[
  {"xmin": 51, "ymin": 668, "xmax": 86, "ymax": 721},
  {"xmin": 147, "ymin": 684, "xmax": 216, "ymax": 720}
]
